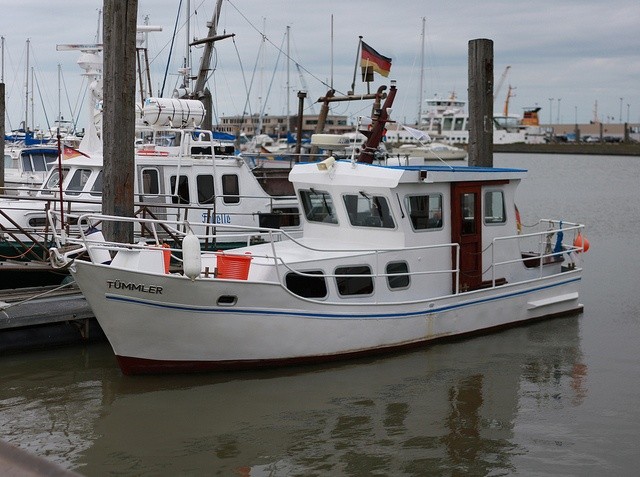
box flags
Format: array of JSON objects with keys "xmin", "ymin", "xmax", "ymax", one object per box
[{"xmin": 358, "ymin": 39, "xmax": 392, "ymax": 78}]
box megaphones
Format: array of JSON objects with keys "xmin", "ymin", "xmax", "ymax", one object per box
[{"xmin": 316, "ymin": 154, "xmax": 335, "ymax": 171}]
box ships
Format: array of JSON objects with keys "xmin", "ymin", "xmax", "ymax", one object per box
[
  {"xmin": 420, "ymin": 96, "xmax": 544, "ymax": 147},
  {"xmin": 210, "ymin": 111, "xmax": 359, "ymax": 139}
]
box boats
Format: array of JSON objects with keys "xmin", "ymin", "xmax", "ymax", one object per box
[
  {"xmin": 48, "ymin": 150, "xmax": 585, "ymax": 362},
  {"xmin": 0, "ymin": 145, "xmax": 422, "ymax": 331},
  {"xmin": 389, "ymin": 143, "xmax": 467, "ymax": 160},
  {"xmin": 1, "ymin": 0, "xmax": 431, "ymax": 146},
  {"xmin": 559, "ymin": 123, "xmax": 639, "ymax": 143}
]
